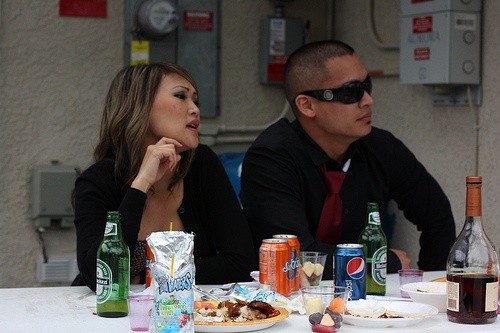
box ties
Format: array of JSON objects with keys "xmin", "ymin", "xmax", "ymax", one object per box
[{"xmin": 316, "ymin": 159, "xmax": 346, "ymax": 246}]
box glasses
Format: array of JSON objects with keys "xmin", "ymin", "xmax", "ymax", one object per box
[{"xmin": 293, "ymin": 74, "xmax": 372, "ymax": 105}]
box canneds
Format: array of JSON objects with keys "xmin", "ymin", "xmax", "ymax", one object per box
[
  {"xmin": 272, "ymin": 235, "xmax": 301, "ymax": 291},
  {"xmin": 259, "ymin": 239, "xmax": 292, "ymax": 297},
  {"xmin": 146, "ymin": 242, "xmax": 156, "ymax": 288},
  {"xmin": 332, "ymin": 244, "xmax": 366, "ymax": 301}
]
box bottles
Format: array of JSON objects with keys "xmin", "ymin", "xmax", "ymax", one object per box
[
  {"xmin": 359, "ymin": 203, "xmax": 387, "ymax": 297},
  {"xmin": 96, "ymin": 212, "xmax": 130, "ymax": 318}
]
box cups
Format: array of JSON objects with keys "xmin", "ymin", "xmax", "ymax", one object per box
[
  {"xmin": 299, "ymin": 252, "xmax": 327, "ymax": 289},
  {"xmin": 126, "ymin": 295, "xmax": 154, "ymax": 331},
  {"xmin": 301, "ymin": 286, "xmax": 349, "ymax": 333},
  {"xmin": 447, "ymin": 176, "xmax": 499, "ymax": 324},
  {"xmin": 398, "ymin": 270, "xmax": 423, "ymax": 298}
]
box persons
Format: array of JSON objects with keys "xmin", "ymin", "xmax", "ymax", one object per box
[
  {"xmin": 70, "ymin": 61, "xmax": 259, "ymax": 292},
  {"xmin": 240, "ymin": 39, "xmax": 456, "ymax": 280}
]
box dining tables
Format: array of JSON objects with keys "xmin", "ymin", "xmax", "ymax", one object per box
[{"xmin": 0, "ymin": 270, "xmax": 499, "ymax": 332}]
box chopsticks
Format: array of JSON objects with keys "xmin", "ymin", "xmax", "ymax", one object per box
[{"xmin": 194, "ymin": 287, "xmax": 220, "ymax": 303}]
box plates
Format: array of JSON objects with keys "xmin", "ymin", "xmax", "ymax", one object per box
[
  {"xmin": 193, "ymin": 300, "xmax": 292, "ymax": 333},
  {"xmin": 400, "ymin": 282, "xmax": 446, "ymax": 313},
  {"xmin": 343, "ymin": 300, "xmax": 439, "ymax": 328}
]
